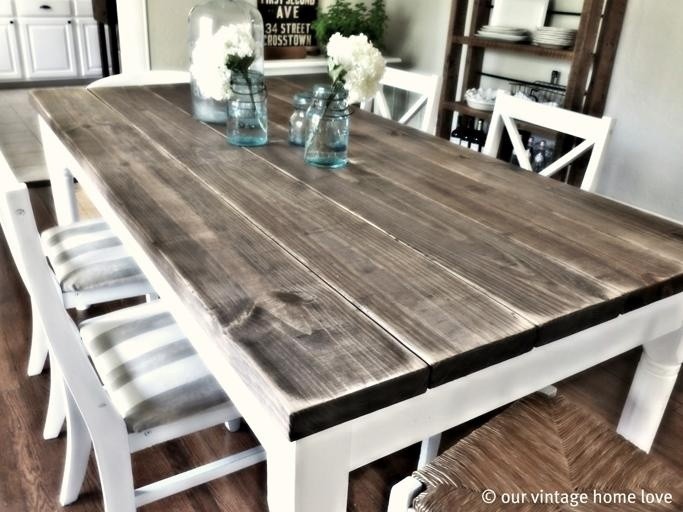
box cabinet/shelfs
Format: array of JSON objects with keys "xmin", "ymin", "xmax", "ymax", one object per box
[
  {"xmin": 435, "ymin": 0, "xmax": 627, "ymax": 198},
  {"xmin": 2, "ymin": 2, "xmax": 116, "ymax": 79}
]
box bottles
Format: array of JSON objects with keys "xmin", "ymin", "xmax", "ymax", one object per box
[
  {"xmin": 448, "ymin": 116, "xmax": 551, "ymax": 174},
  {"xmin": 190, "ymin": 0, "xmax": 351, "ymax": 169}
]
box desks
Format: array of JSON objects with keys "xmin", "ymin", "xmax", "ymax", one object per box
[{"xmin": 26, "ymin": 77, "xmax": 683, "ymax": 511}]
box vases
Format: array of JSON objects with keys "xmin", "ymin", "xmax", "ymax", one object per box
[
  {"xmin": 303, "ymin": 80, "xmax": 350, "ymax": 170},
  {"xmin": 226, "ymin": 71, "xmax": 269, "ymax": 147}
]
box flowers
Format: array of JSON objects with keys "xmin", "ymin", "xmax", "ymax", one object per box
[
  {"xmin": 209, "ymin": 19, "xmax": 266, "ymax": 128},
  {"xmin": 301, "ymin": 31, "xmax": 387, "ymax": 163}
]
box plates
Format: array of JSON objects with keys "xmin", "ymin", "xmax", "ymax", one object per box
[
  {"xmin": 466, "ymin": 97, "xmax": 497, "ymax": 111},
  {"xmin": 472, "ymin": 21, "xmax": 580, "ymax": 49}
]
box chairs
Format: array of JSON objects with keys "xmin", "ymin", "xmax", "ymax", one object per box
[
  {"xmin": 359, "ymin": 68, "xmax": 613, "ymax": 197},
  {"xmin": 0, "ymin": 150, "xmax": 273, "ymax": 511}
]
